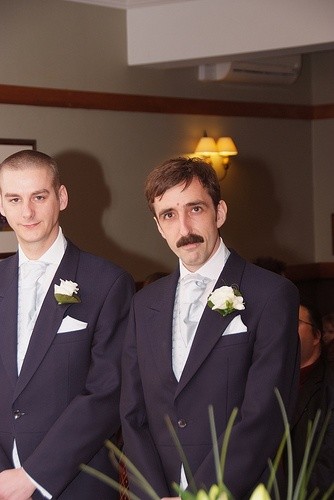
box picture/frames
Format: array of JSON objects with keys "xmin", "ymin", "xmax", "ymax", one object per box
[{"xmin": 0, "ymin": 138, "xmax": 37, "ymax": 232}]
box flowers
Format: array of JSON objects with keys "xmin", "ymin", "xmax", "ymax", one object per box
[
  {"xmin": 207, "ymin": 285, "xmax": 246, "ymax": 317},
  {"xmin": 54, "ymin": 278, "xmax": 81, "ymax": 305}
]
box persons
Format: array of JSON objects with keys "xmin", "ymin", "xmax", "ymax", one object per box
[
  {"xmin": 0, "ymin": 150, "xmax": 137, "ymax": 500},
  {"xmin": 118, "ymin": 156, "xmax": 302, "ymax": 500},
  {"xmin": 132, "ymin": 255, "xmax": 334, "ymax": 499}
]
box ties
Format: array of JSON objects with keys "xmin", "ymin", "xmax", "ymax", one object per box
[
  {"xmin": 172, "ymin": 274, "xmax": 210, "ymax": 383},
  {"xmin": 18, "ymin": 261, "xmax": 48, "ymax": 377}
]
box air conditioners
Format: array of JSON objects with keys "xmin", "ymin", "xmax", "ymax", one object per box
[{"xmin": 198, "ymin": 54, "xmax": 302, "ymax": 85}]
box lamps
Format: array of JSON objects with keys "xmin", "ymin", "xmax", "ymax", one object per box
[{"xmin": 195, "ymin": 130, "xmax": 238, "ymax": 181}]
